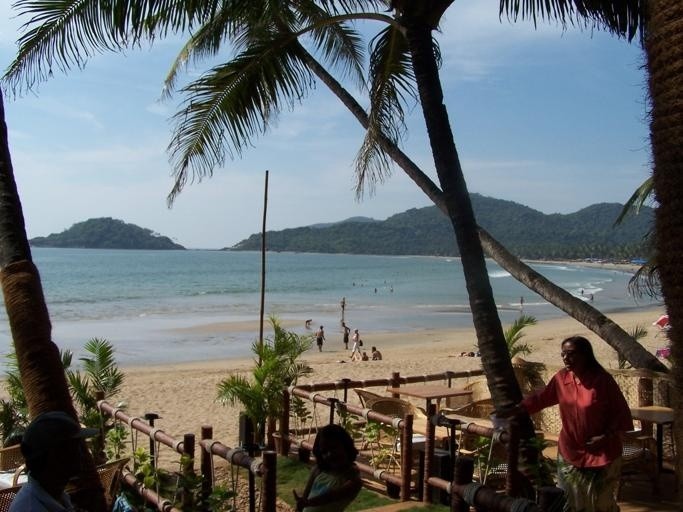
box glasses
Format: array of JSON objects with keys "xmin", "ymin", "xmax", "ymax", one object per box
[{"xmin": 561, "ymin": 352, "xmax": 577, "ymax": 357}]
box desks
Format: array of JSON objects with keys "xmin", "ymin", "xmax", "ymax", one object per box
[{"xmin": 629, "ymin": 404, "xmax": 674, "ymax": 505}]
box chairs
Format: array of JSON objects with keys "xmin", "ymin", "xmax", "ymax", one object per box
[{"xmin": 349, "ymin": 381, "xmax": 501, "ymax": 489}]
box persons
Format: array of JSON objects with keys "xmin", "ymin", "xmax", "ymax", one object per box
[
  {"xmin": 7, "ymin": 412, "xmax": 98, "ymax": 512},
  {"xmin": 591, "ymin": 295, "xmax": 593, "ymax": 301},
  {"xmin": 305, "ymin": 280, "xmax": 393, "ymax": 361},
  {"xmin": 514, "ymin": 335, "xmax": 634, "ymax": 512},
  {"xmin": 520, "ymin": 296, "xmax": 524, "ymax": 305},
  {"xmin": 294, "ymin": 423, "xmax": 364, "ymax": 512},
  {"xmin": 461, "ymin": 351, "xmax": 475, "ymax": 357},
  {"xmin": 582, "ymin": 289, "xmax": 584, "ymax": 294}
]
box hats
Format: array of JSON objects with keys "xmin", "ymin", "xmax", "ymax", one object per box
[{"xmin": 22, "ymin": 412, "xmax": 99, "ymax": 446}]
